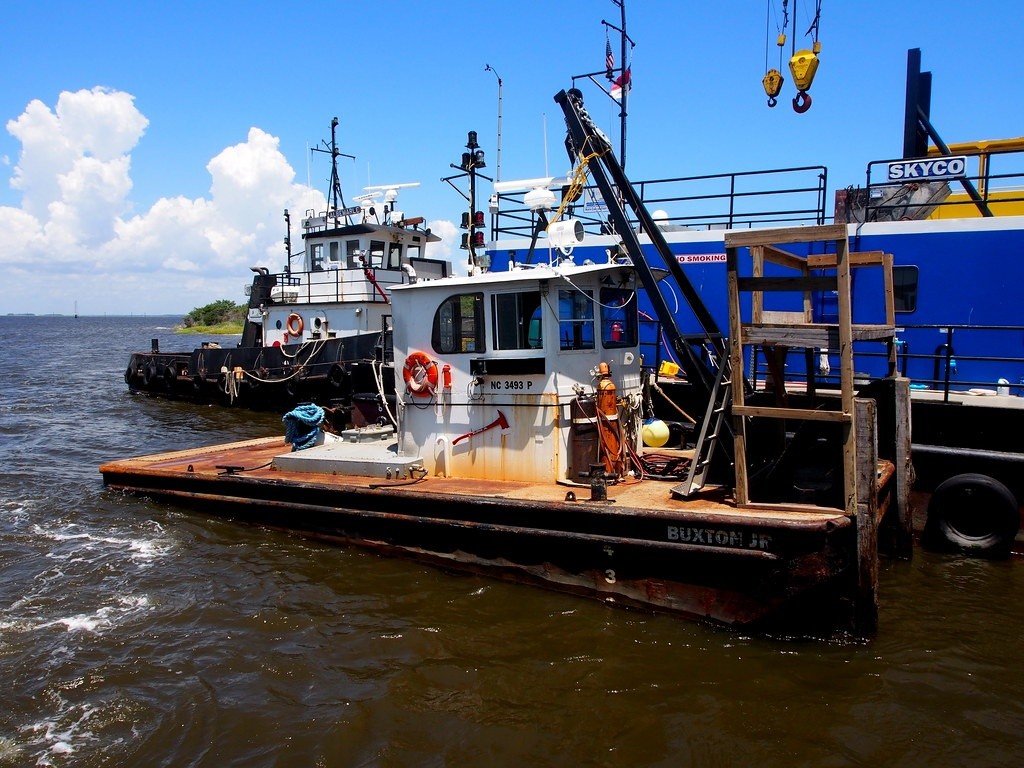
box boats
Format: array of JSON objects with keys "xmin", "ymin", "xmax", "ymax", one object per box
[
  {"xmin": 124, "ymin": 118, "xmax": 465, "ymax": 409},
  {"xmin": 466, "ymin": 0, "xmax": 1024, "ymax": 560},
  {"xmin": 101, "ymin": 81, "xmax": 920, "ymax": 650}
]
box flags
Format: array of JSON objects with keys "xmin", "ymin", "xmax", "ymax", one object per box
[
  {"xmin": 606, "ymin": 26, "xmax": 613, "ymax": 70},
  {"xmin": 611, "ymin": 65, "xmax": 631, "ymax": 99}
]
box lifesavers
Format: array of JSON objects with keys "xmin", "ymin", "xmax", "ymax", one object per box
[
  {"xmin": 403, "ymin": 352, "xmax": 438, "ymax": 398},
  {"xmin": 925, "ymin": 471, "xmax": 1022, "ymax": 554},
  {"xmin": 123, "ymin": 360, "xmax": 352, "ymax": 398},
  {"xmin": 287, "ymin": 313, "xmax": 303, "ymax": 337}
]
